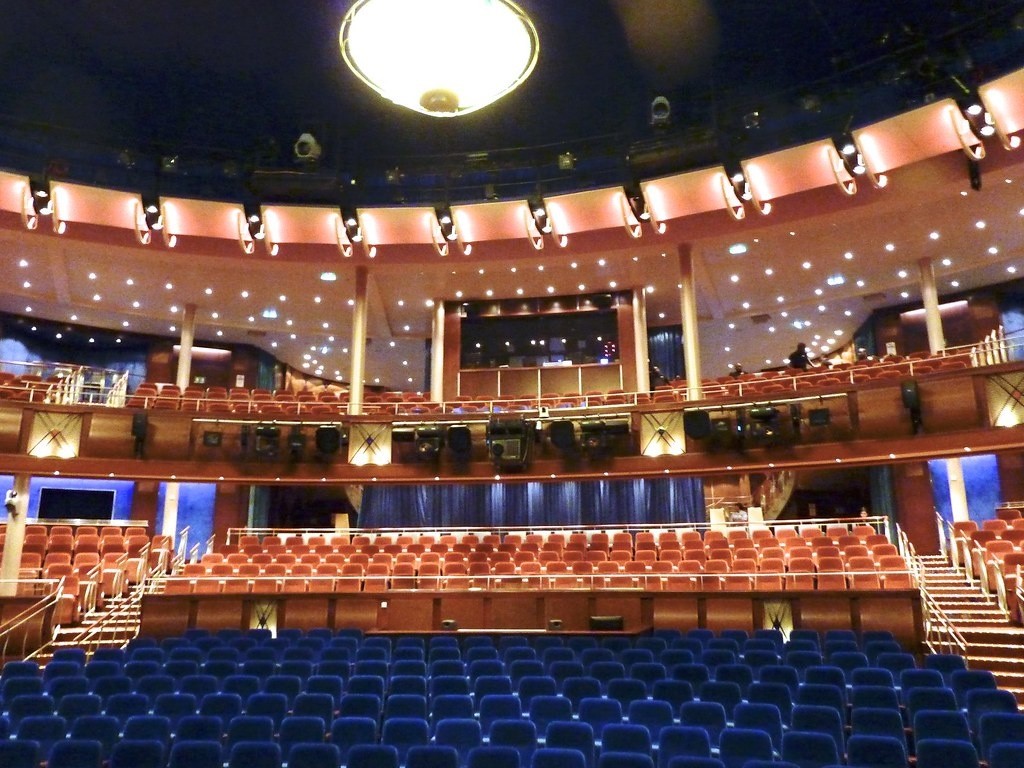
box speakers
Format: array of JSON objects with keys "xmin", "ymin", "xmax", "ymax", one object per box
[
  {"xmin": 902, "ymin": 379, "xmax": 919, "ymax": 409},
  {"xmin": 316, "ymin": 426, "xmax": 340, "ymax": 454},
  {"xmin": 131, "ymin": 412, "xmax": 148, "ymax": 437},
  {"xmin": 684, "ymin": 410, "xmax": 712, "ymax": 440},
  {"xmin": 447, "ymin": 426, "xmax": 471, "ymax": 453},
  {"xmin": 551, "ymin": 420, "xmax": 575, "ymax": 449}
]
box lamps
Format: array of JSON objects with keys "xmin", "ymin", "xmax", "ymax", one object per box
[
  {"xmin": 808, "ymin": 401, "xmax": 831, "ymax": 426},
  {"xmin": 711, "ymin": 411, "xmax": 733, "ymax": 435},
  {"xmin": 202, "ymin": 424, "xmax": 221, "ymax": 446},
  {"xmin": 288, "ymin": 426, "xmax": 307, "ymax": 448}
]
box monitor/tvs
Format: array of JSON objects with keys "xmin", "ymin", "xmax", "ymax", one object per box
[{"xmin": 590, "ymin": 617, "xmax": 625, "ymax": 631}]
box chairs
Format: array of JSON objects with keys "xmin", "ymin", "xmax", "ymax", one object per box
[
  {"xmin": 0, "ymin": 372, "xmax": 71, "ymax": 402},
  {"xmin": 127, "ymin": 347, "xmax": 978, "ymax": 414},
  {"xmin": 0, "ymin": 514, "xmax": 1024, "ymax": 768}
]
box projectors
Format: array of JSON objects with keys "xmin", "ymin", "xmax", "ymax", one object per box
[{"xmin": 491, "ymin": 439, "xmax": 521, "ymax": 459}]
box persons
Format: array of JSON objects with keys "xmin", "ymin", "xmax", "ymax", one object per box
[
  {"xmin": 729, "ymin": 363, "xmax": 748, "ymax": 380},
  {"xmin": 650, "ymin": 366, "xmax": 670, "ymax": 397},
  {"xmin": 820, "ymin": 353, "xmax": 832, "ymax": 367},
  {"xmin": 730, "ymin": 503, "xmax": 748, "ymax": 527},
  {"xmin": 789, "ymin": 343, "xmax": 817, "ymax": 372},
  {"xmin": 855, "ymin": 347, "xmax": 870, "ymax": 366}
]
